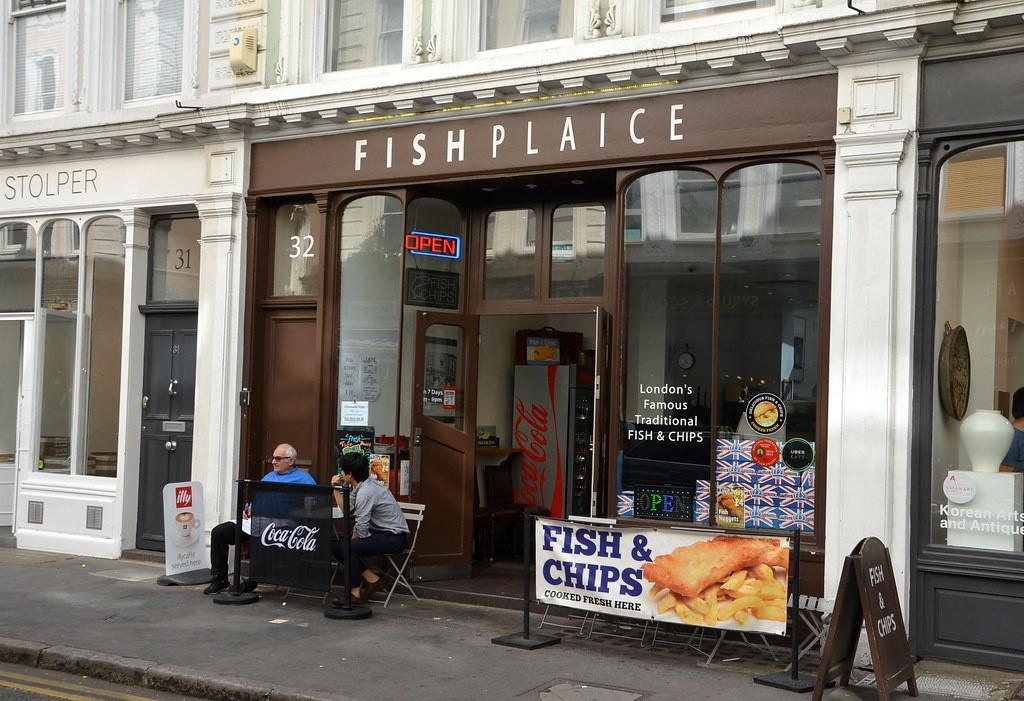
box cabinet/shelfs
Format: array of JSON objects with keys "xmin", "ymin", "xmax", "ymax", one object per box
[{"xmin": 511, "ymin": 365, "xmax": 595, "ymax": 539}]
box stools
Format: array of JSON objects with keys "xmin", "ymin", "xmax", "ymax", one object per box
[{"xmin": 473, "ymin": 503, "xmax": 541, "ymax": 567}]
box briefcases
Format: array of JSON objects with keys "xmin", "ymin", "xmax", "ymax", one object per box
[{"xmin": 515, "ymin": 326, "xmax": 583, "ymax": 364}]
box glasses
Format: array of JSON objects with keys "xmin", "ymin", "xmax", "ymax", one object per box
[{"xmin": 272, "ymin": 456, "xmax": 290, "ymax": 462}]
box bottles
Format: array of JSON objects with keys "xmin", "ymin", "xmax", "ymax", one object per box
[{"xmin": 574, "ymin": 399, "xmax": 593, "ymax": 422}]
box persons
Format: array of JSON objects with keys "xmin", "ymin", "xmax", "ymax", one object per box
[
  {"xmin": 203, "ymin": 443, "xmax": 327, "ymax": 594},
  {"xmin": 999, "ymin": 386, "xmax": 1024, "ymax": 472},
  {"xmin": 332, "ymin": 453, "xmax": 410, "ymax": 606}
]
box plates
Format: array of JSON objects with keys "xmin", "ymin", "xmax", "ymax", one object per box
[
  {"xmin": 938, "ymin": 324, "xmax": 972, "ymax": 422},
  {"xmin": 171, "ymin": 527, "xmax": 200, "ymax": 547}
]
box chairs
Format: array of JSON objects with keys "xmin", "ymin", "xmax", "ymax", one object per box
[{"xmin": 355, "ymin": 501, "xmax": 425, "ymax": 607}]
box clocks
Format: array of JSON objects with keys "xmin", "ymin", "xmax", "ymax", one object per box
[{"xmin": 667, "ymin": 343, "xmax": 696, "ymax": 372}]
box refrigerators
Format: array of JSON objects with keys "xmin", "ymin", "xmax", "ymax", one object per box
[{"xmin": 511, "ymin": 364, "xmax": 594, "ymax": 521}]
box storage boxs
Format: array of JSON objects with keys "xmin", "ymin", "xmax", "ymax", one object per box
[{"xmin": 515, "ymin": 329, "xmax": 577, "ymax": 365}]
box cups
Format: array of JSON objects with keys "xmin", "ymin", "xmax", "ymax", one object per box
[
  {"xmin": 175, "ymin": 512, "xmax": 201, "ymax": 538},
  {"xmin": 303, "ymin": 496, "xmax": 318, "ymax": 512}
]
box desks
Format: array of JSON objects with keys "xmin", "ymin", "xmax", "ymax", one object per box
[
  {"xmin": 946, "ymin": 470, "xmax": 1023, "ymax": 553},
  {"xmin": 470, "ymin": 447, "xmax": 523, "ymax": 507},
  {"xmin": 281, "ymin": 507, "xmax": 344, "ymax": 603}
]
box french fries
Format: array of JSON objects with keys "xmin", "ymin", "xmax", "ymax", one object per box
[{"xmin": 649, "ymin": 563, "xmax": 788, "ymax": 626}]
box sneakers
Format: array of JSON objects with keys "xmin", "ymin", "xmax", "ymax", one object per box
[
  {"xmin": 331, "ymin": 593, "xmax": 362, "ymax": 605},
  {"xmin": 228, "ymin": 578, "xmax": 256, "ymax": 594},
  {"xmin": 204, "ymin": 576, "xmax": 229, "ymax": 595},
  {"xmin": 360, "ymin": 576, "xmax": 384, "ymax": 599}
]
